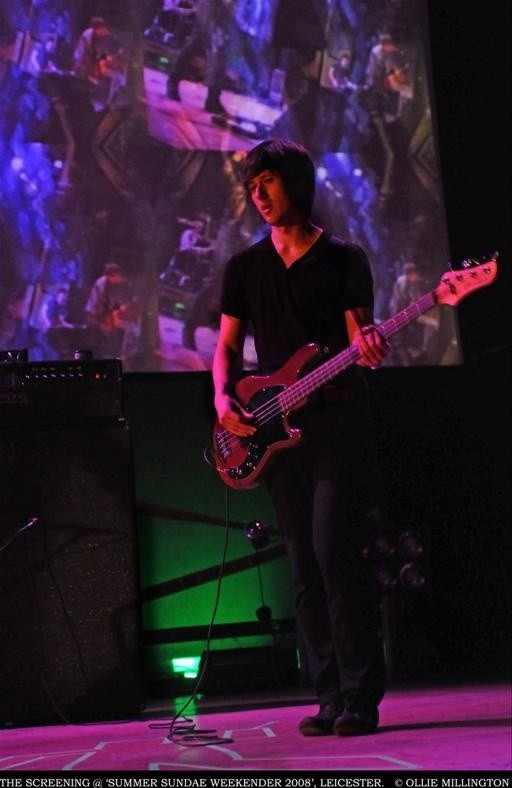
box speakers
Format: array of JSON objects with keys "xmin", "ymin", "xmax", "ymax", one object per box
[{"xmin": 0, "ymin": 424, "xmax": 144, "ymax": 728}]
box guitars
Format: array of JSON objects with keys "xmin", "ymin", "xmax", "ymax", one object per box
[{"xmin": 211, "ymin": 251, "xmax": 499, "ymax": 490}]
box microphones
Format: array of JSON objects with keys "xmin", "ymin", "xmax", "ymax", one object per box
[{"xmin": 18, "ymin": 515, "xmax": 40, "ymax": 534}]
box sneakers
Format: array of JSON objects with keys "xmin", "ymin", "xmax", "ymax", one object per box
[
  {"xmin": 332, "ymin": 700, "xmax": 379, "ymax": 736},
  {"xmin": 299, "ymin": 701, "xmax": 345, "ymax": 736}
]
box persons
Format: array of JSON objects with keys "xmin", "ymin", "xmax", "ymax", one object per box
[
  {"xmin": 212, "ymin": 136, "xmax": 390, "ymax": 737},
  {"xmin": 0, "ymin": 0, "xmax": 460, "ymax": 367}
]
